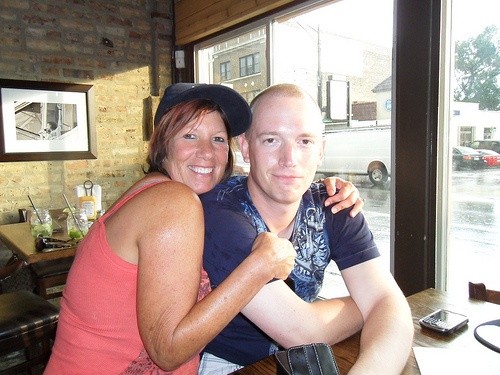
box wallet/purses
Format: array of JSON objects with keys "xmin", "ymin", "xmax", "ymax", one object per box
[{"xmin": 275, "ymin": 343, "xmax": 340, "ymax": 375}]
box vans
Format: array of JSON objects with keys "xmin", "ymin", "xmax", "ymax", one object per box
[{"xmin": 468, "ymin": 139, "xmax": 500, "ymax": 154}]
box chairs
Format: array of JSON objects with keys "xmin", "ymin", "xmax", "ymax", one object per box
[
  {"xmin": 18, "ymin": 209, "xmax": 71, "ymax": 302},
  {"xmin": 0, "ymin": 290, "xmax": 58, "ymax": 375}
]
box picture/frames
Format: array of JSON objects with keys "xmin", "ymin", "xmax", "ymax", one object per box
[{"xmin": 0, "ymin": 78, "xmax": 99, "ymax": 162}]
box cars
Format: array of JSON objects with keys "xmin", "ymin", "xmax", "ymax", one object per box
[
  {"xmin": 475, "ymin": 148, "xmax": 500, "ymax": 168},
  {"xmin": 451, "ymin": 145, "xmax": 484, "ymax": 171}
]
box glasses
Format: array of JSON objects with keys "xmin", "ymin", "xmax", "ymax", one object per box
[{"xmin": 34, "ymin": 234, "xmax": 75, "ymax": 251}]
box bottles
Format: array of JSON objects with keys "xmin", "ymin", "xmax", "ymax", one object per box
[
  {"xmin": 96, "ymin": 210, "xmax": 105, "ymax": 219},
  {"xmin": 67, "ymin": 208, "xmax": 89, "ymax": 241},
  {"xmin": 30, "ymin": 208, "xmax": 53, "ymax": 241}
]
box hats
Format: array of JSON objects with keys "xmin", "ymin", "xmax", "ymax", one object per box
[{"xmin": 154, "ymin": 83, "xmax": 252, "ymax": 137}]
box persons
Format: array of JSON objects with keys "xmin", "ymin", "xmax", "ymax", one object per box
[
  {"xmin": 42, "ymin": 82, "xmax": 298, "ymax": 375},
  {"xmin": 46, "ymin": 121, "xmax": 57, "ymax": 132},
  {"xmin": 197, "ymin": 84, "xmax": 416, "ymax": 375}
]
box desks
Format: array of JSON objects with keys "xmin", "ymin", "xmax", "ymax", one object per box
[
  {"xmin": 224, "ymin": 286, "xmax": 500, "ymax": 375},
  {"xmin": 0, "ymin": 219, "xmax": 81, "ymax": 368}
]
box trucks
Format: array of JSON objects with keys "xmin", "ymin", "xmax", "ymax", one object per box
[{"xmin": 316, "ymin": 123, "xmax": 391, "ymax": 186}]
